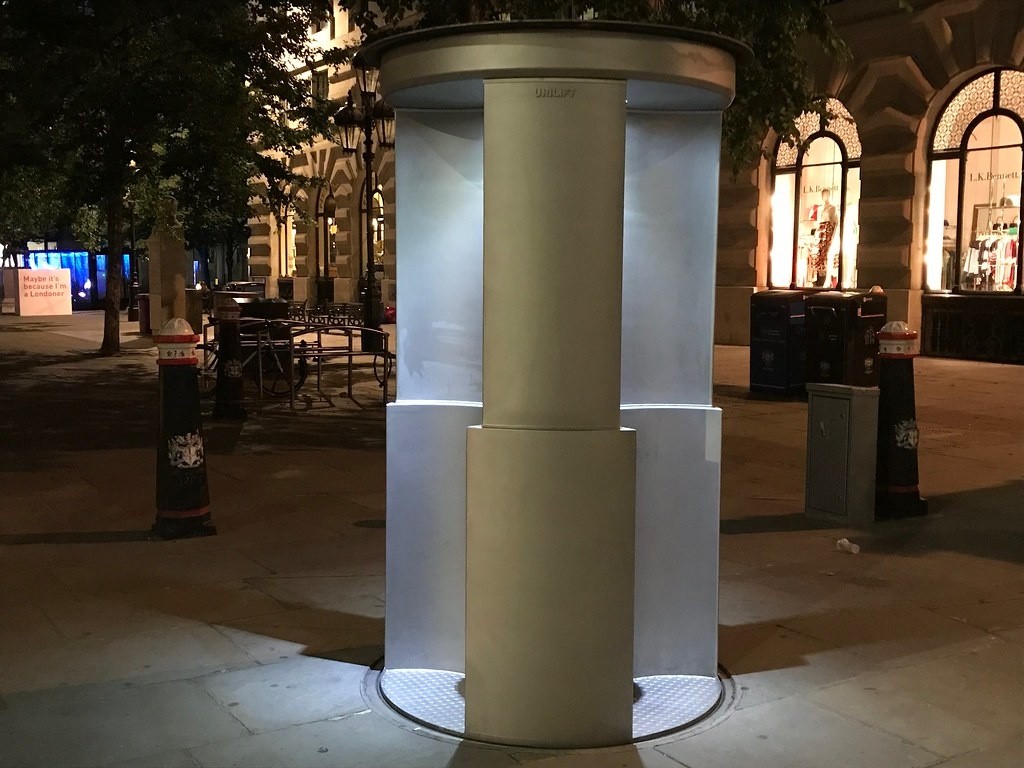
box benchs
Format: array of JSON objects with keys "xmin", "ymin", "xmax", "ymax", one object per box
[{"xmin": 286, "ymin": 298, "xmax": 365, "ymax": 327}]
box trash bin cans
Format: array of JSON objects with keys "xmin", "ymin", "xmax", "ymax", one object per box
[
  {"xmin": 225, "ymin": 281, "xmax": 264, "ymax": 299},
  {"xmin": 749, "ymin": 289, "xmax": 809, "ymax": 399},
  {"xmin": 230, "ymin": 296, "xmax": 290, "ymax": 370},
  {"xmin": 134, "ymin": 293, "xmax": 152, "ymax": 334},
  {"xmin": 803, "ymin": 285, "xmax": 887, "ymax": 388}
]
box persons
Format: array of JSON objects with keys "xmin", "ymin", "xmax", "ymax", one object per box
[
  {"xmin": 812, "ymin": 189, "xmax": 838, "ymax": 287},
  {"xmin": 200, "ymin": 281, "xmax": 212, "ymax": 315}
]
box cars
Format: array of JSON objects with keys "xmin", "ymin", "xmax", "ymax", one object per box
[{"xmin": 220, "ymin": 281, "xmax": 264, "ymax": 304}]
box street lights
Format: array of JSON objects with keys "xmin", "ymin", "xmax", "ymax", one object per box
[
  {"xmin": 334, "ymin": 41, "xmax": 396, "ymax": 353},
  {"xmin": 128, "ymin": 160, "xmax": 140, "ymax": 323}
]
